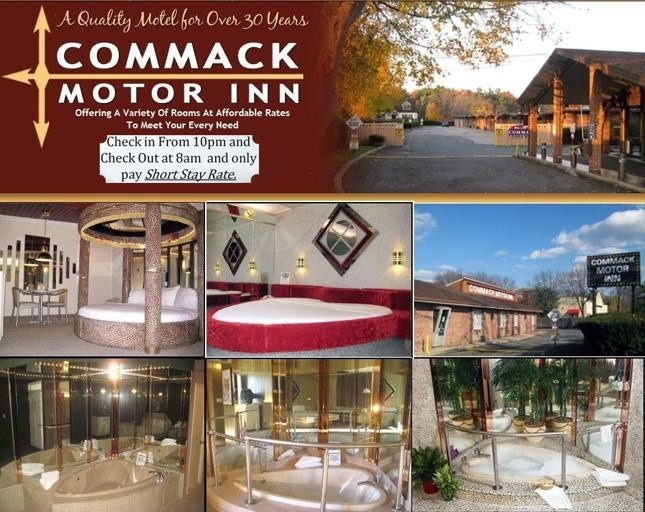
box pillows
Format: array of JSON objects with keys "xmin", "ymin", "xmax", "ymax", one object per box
[
  {"xmin": 128, "ymin": 288, "xmax": 145, "ymax": 305},
  {"xmin": 161, "ymin": 286, "xmax": 179, "ymax": 306},
  {"xmin": 173, "ymin": 287, "xmax": 197, "ymax": 310}
]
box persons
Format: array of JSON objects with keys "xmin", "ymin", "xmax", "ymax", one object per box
[
  {"xmin": 617, "ymin": 376, "xmax": 623, "ymax": 405},
  {"xmin": 439, "ymin": 315, "xmax": 447, "ymax": 331},
  {"xmin": 622, "ymin": 376, "xmax": 631, "ymax": 407}
]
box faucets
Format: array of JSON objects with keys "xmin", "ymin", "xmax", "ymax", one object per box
[
  {"xmin": 354, "ymin": 479, "xmax": 378, "ymax": 486},
  {"xmin": 472, "ymin": 448, "xmax": 491, "ymax": 458},
  {"xmin": 148, "ymin": 469, "xmax": 165, "ymax": 484}
]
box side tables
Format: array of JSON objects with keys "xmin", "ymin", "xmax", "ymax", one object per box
[{"xmin": 30, "ymin": 291, "xmax": 52, "ymax": 324}]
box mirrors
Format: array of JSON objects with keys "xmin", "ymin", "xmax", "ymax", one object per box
[
  {"xmin": 207, "ymin": 204, "xmax": 276, "ymax": 309},
  {"xmin": 204, "ymin": 358, "xmax": 409, "ymax": 500},
  {"xmin": 0, "ymin": 356, "xmax": 194, "ymax": 489},
  {"xmin": 0, "ymin": 234, "xmax": 76, "ymax": 293},
  {"xmin": 585, "ymin": 358, "xmax": 634, "ymax": 472},
  {"xmin": 429, "ymin": 357, "xmax": 492, "ymax": 463}
]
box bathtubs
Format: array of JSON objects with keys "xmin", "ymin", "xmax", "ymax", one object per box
[
  {"xmin": 459, "ymin": 440, "xmax": 597, "ymax": 481},
  {"xmin": 0, "ymin": 436, "xmax": 146, "ymax": 482},
  {"xmin": 52, "ymin": 457, "xmax": 156, "ymax": 498},
  {"xmin": 232, "ymin": 463, "xmax": 387, "ymax": 508}
]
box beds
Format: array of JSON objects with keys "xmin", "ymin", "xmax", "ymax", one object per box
[
  {"xmin": 207, "ymin": 284, "xmax": 411, "ymax": 354},
  {"xmin": 77, "ymin": 302, "xmax": 199, "ymax": 349},
  {"xmin": 207, "ymin": 288, "xmax": 242, "ymax": 302}
]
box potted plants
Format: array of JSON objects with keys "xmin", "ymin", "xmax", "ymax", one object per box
[
  {"xmin": 411, "ymin": 446, "xmax": 460, "ymax": 502},
  {"xmin": 490, "ymin": 358, "xmax": 591, "ymax": 444},
  {"xmin": 432, "ymin": 357, "xmax": 487, "ymax": 436}
]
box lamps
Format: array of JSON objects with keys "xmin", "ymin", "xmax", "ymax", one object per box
[
  {"xmin": 393, "ymin": 248, "xmax": 402, "ymax": 265},
  {"xmin": 35, "ymin": 210, "xmax": 52, "ymax": 261},
  {"xmin": 249, "ymin": 261, "xmax": 255, "ymax": 270},
  {"xmin": 297, "ymin": 258, "xmax": 303, "ymax": 267},
  {"xmin": 216, "ymin": 264, "xmax": 220, "ymax": 270}
]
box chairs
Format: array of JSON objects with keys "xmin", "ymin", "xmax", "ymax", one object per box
[
  {"xmin": 10, "ymin": 287, "xmax": 41, "ymax": 327},
  {"xmin": 42, "ymin": 289, "xmax": 68, "ymax": 325}
]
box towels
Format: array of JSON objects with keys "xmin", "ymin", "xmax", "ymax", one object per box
[
  {"xmin": 599, "ymin": 424, "xmax": 614, "ymax": 444},
  {"xmin": 592, "ymin": 468, "xmax": 627, "ymax": 488},
  {"xmin": 536, "ymin": 487, "xmax": 570, "ymax": 510}
]
